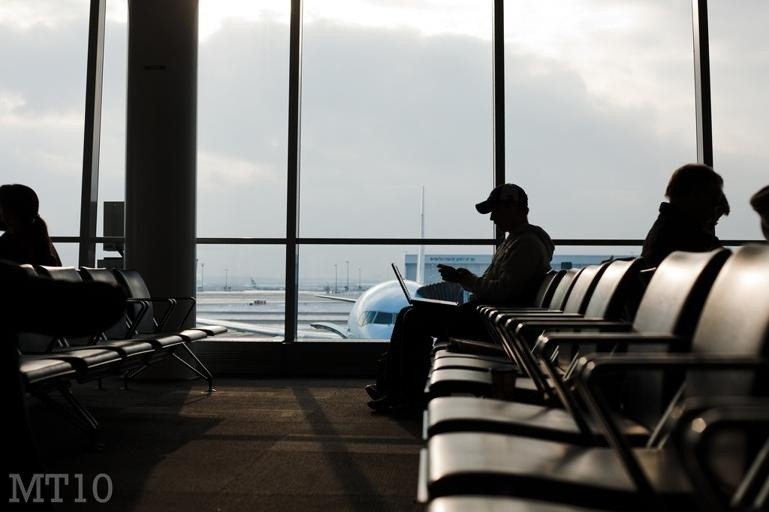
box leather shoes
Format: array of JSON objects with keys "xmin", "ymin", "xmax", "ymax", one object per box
[{"xmin": 364, "ymin": 381, "xmax": 424, "ymax": 418}]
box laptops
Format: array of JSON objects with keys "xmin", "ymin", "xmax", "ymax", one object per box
[{"xmin": 391, "ymin": 261, "xmax": 461, "ymax": 310}]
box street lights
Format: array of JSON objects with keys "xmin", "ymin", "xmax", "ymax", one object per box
[
  {"xmin": 333, "ymin": 260, "xmax": 363, "ymax": 293},
  {"xmin": 197, "ymin": 258, "xmax": 230, "ymax": 291}
]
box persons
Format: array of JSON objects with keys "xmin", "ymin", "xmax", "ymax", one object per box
[
  {"xmin": 366, "ymin": 186, "xmax": 555, "ymax": 407},
  {"xmin": 0, "ymin": 184, "xmax": 62, "ymax": 267},
  {"xmin": 640, "ymin": 159, "xmax": 734, "ymax": 264}
]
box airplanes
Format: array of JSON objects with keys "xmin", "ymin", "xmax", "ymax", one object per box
[{"xmin": 195, "ymin": 186, "xmax": 432, "ymax": 343}]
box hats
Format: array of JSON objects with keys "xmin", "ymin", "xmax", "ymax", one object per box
[{"xmin": 475, "ymin": 183, "xmax": 529, "ymax": 214}]
